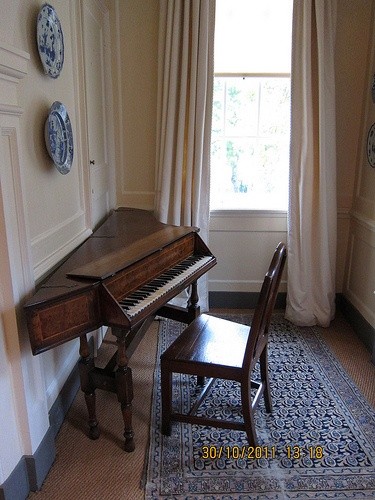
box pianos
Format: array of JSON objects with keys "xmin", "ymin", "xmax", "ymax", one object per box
[{"xmin": 25, "ymin": 207, "xmax": 219, "ymax": 452}]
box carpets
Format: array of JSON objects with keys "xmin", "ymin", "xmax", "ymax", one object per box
[{"xmin": 142, "ymin": 311, "xmax": 374, "ymax": 500}]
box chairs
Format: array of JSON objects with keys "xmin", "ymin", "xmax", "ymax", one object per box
[{"xmin": 160, "ymin": 243, "xmax": 287, "ymax": 447}]
